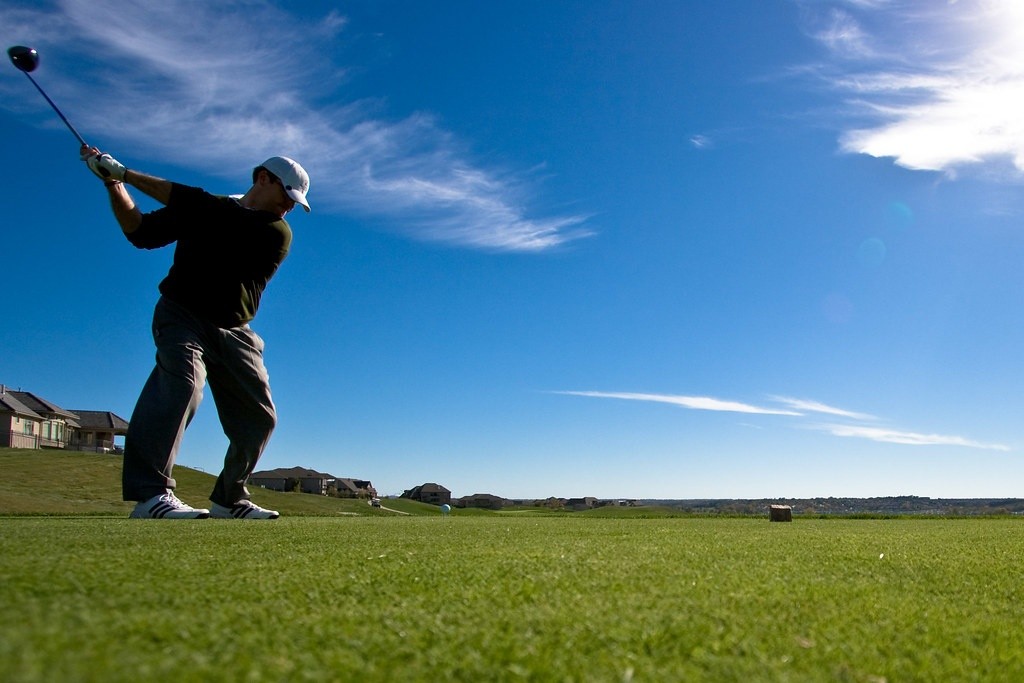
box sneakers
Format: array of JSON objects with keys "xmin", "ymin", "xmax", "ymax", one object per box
[
  {"xmin": 261, "ymin": 156, "xmax": 311, "ymax": 212},
  {"xmin": 129, "ymin": 492, "xmax": 209, "ymax": 519},
  {"xmin": 210, "ymin": 499, "xmax": 279, "ymax": 519}
]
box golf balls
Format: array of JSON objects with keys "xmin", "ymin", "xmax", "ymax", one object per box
[{"xmin": 441, "ymin": 503, "xmax": 451, "ymax": 513}]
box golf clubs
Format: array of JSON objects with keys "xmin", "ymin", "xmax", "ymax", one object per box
[{"xmin": 8, "ymin": 46, "xmax": 89, "ymax": 150}]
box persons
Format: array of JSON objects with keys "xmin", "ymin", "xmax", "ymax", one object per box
[{"xmin": 76, "ymin": 140, "xmax": 312, "ymax": 520}]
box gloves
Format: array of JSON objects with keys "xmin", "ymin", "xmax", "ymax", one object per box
[{"xmin": 80, "ymin": 147, "xmax": 126, "ymax": 187}]
